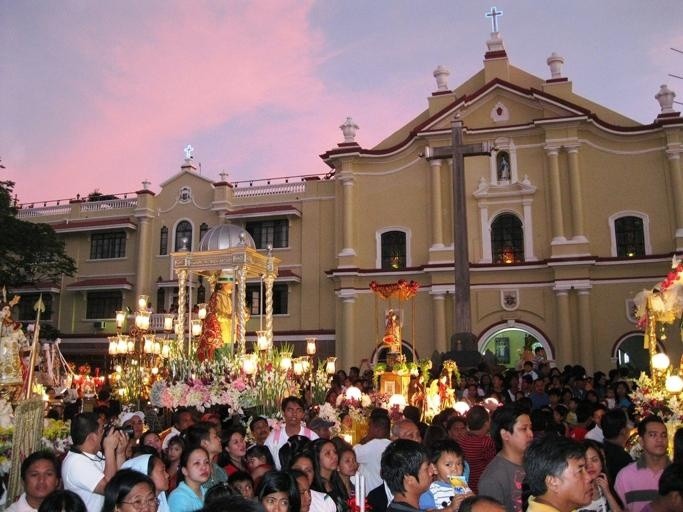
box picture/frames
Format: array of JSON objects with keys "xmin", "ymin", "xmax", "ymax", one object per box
[{"xmin": 495, "ymin": 338, "xmax": 511, "ymax": 364}]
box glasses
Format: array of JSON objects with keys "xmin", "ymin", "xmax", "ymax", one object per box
[{"xmin": 119, "ymin": 498, "xmax": 157, "ymax": 511}]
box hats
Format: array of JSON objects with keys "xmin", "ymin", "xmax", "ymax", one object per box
[
  {"xmin": 120, "ymin": 411, "xmax": 145, "ymax": 427},
  {"xmin": 309, "ymin": 417, "xmax": 335, "ymax": 429}
]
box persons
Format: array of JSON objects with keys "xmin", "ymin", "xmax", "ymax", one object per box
[
  {"xmin": 0, "ymin": 302, "xmax": 31, "ymax": 404},
  {"xmin": 197, "ymin": 274, "xmax": 250, "ymax": 361},
  {"xmin": 0, "ymin": 347, "xmax": 683, "ymax": 512}
]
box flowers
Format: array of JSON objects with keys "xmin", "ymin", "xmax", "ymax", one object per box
[
  {"xmin": 0, "ymin": 418, "xmax": 74, "ymax": 478},
  {"xmin": 624, "ymin": 366, "xmax": 683, "ymax": 463}
]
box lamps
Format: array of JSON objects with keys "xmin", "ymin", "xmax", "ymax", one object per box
[
  {"xmin": 243, "ymin": 331, "xmax": 337, "ymax": 382},
  {"xmin": 108, "ymin": 294, "xmax": 205, "ymax": 410},
  {"xmin": 651, "ymin": 351, "xmax": 682, "ymax": 396}
]
